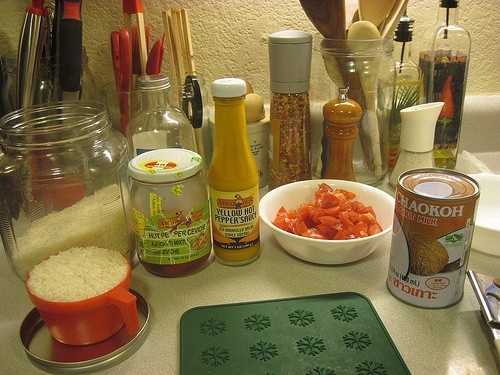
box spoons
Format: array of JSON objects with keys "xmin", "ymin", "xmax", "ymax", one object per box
[{"xmin": 298, "ymin": 0, "xmax": 408, "ymax": 176}]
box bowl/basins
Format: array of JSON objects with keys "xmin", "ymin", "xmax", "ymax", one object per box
[{"xmin": 258, "ymin": 178, "xmax": 395, "ymax": 265}]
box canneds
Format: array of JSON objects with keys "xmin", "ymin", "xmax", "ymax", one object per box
[
  {"xmin": 386, "ymin": 168, "xmax": 480, "ymax": 309},
  {"xmin": 128, "ymin": 149, "xmax": 211, "ymax": 278}
]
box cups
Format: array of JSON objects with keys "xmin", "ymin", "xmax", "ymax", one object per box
[{"xmin": 24, "ymin": 249, "xmax": 143, "ymax": 347}]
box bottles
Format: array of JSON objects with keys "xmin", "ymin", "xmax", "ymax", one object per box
[
  {"xmin": 391, "ymin": 17, "xmax": 423, "ymax": 154},
  {"xmin": 267, "ymin": 31, "xmax": 315, "ymax": 191},
  {"xmin": 320, "ymin": 88, "xmax": 364, "ymax": 183},
  {"xmin": 386, "ymin": 102, "xmax": 445, "ymax": 192},
  {"xmin": 1, "ymin": 100, "xmax": 145, "ymax": 284},
  {"xmin": 124, "ymin": 74, "xmax": 200, "ymax": 160},
  {"xmin": 311, "ymin": 33, "xmax": 403, "ymax": 187},
  {"xmin": 417, "ymin": 0, "xmax": 472, "ymax": 171},
  {"xmin": 205, "ymin": 77, "xmax": 262, "ymax": 266}
]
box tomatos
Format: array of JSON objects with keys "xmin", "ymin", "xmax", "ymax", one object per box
[{"xmin": 271, "ymin": 183, "xmax": 382, "ymax": 241}]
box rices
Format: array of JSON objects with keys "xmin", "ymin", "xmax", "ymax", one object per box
[
  {"xmin": 11, "ymin": 183, "xmax": 136, "ymax": 280},
  {"xmin": 27, "ymin": 246, "xmax": 129, "ymax": 302}
]
